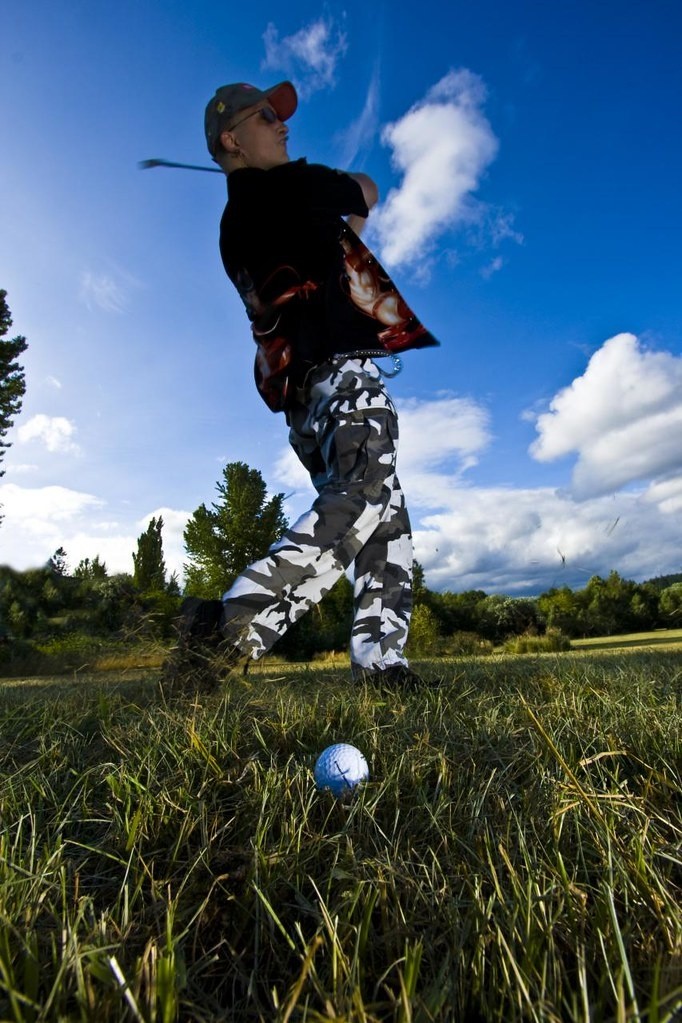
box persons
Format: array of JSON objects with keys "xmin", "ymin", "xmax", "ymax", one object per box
[{"xmin": 169, "ymin": 82, "xmax": 440, "ymax": 704}]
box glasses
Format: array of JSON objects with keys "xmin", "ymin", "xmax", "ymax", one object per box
[{"xmin": 218, "ymin": 106, "xmax": 284, "ymax": 151}]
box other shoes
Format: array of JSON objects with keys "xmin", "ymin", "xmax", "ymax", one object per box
[
  {"xmin": 157, "ymin": 597, "xmax": 248, "ymax": 711},
  {"xmin": 353, "ymin": 666, "xmax": 444, "ymax": 698}
]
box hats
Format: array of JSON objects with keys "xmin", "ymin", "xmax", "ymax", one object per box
[{"xmin": 205, "ymin": 81, "xmax": 297, "ymax": 163}]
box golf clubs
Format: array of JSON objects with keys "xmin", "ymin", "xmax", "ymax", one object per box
[{"xmin": 136, "ymin": 158, "xmax": 224, "ymax": 174}]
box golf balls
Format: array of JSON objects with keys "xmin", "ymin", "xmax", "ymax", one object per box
[{"xmin": 315, "ymin": 741, "xmax": 369, "ymax": 791}]
